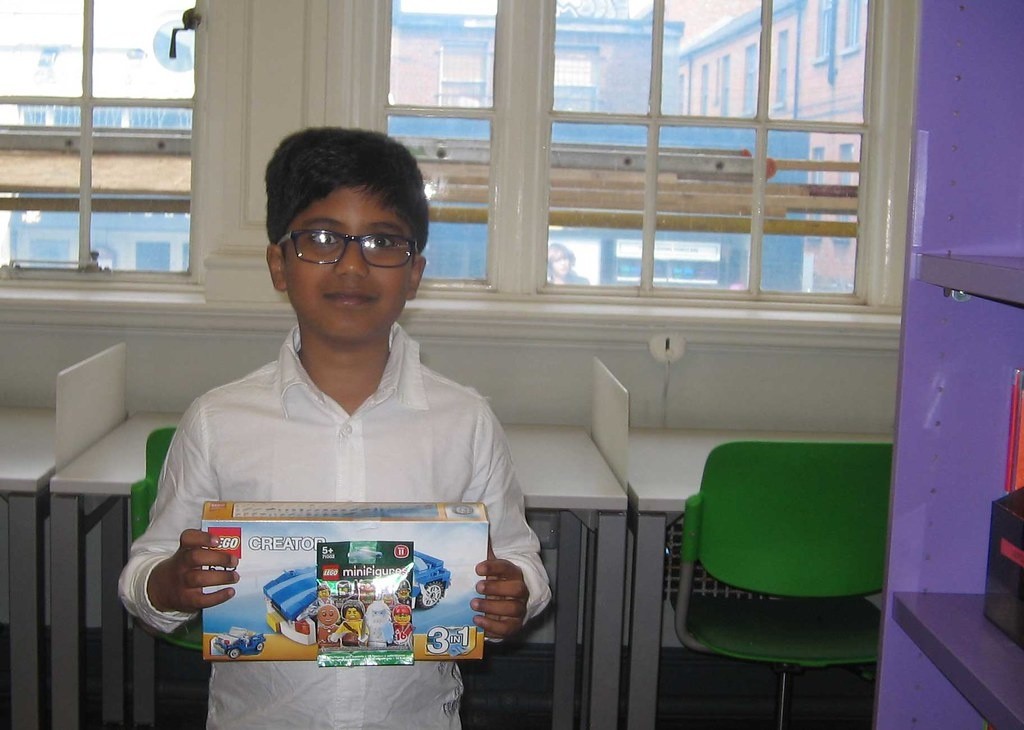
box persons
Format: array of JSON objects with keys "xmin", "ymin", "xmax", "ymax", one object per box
[{"xmin": 119, "ymin": 127, "xmax": 551, "ymax": 730}]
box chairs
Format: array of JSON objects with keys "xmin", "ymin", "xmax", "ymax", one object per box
[
  {"xmin": 672, "ymin": 438, "xmax": 894, "ymax": 730},
  {"xmin": 132, "ymin": 426, "xmax": 204, "ymax": 652}
]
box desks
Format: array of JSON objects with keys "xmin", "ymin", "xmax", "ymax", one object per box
[
  {"xmin": 0, "ymin": 400, "xmax": 56, "ymax": 730},
  {"xmin": 48, "ymin": 411, "xmax": 627, "ymax": 730},
  {"xmin": 624, "ymin": 425, "xmax": 895, "ymax": 730}
]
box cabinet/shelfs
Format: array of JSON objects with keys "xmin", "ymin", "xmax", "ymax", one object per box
[{"xmin": 870, "ymin": 0, "xmax": 1024, "ymax": 730}]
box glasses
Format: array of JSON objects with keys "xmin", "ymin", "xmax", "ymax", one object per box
[{"xmin": 277, "ymin": 229, "xmax": 420, "ymax": 268}]
